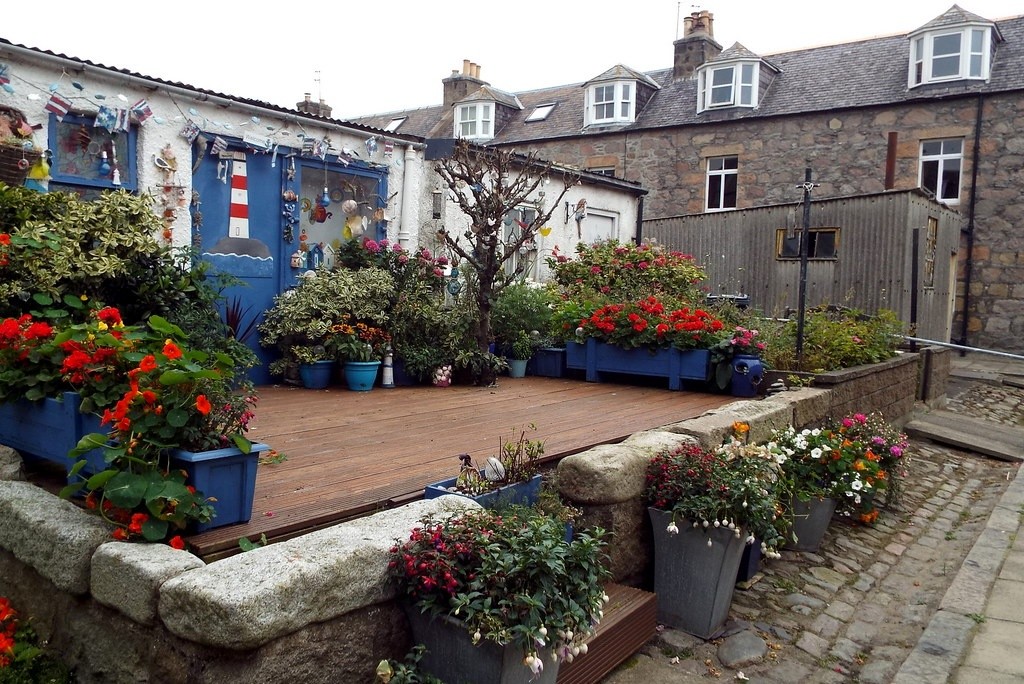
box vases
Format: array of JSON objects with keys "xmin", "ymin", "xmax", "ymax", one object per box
[{"xmin": 0, "ymin": 334, "xmax": 843, "ymax": 684}]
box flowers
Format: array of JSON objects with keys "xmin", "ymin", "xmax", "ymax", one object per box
[
  {"xmin": 389, "ymin": 511, "xmax": 615, "ymax": 674},
  {"xmin": 647, "ymin": 409, "xmax": 912, "ymax": 559},
  {"xmin": 374, "ymin": 645, "xmax": 441, "ymax": 684},
  {"xmin": 0, "ymin": 179, "xmax": 260, "ymax": 551},
  {"xmin": 325, "ymin": 235, "xmax": 761, "ymax": 363}
]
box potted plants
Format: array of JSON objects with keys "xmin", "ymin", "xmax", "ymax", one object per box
[
  {"xmin": 508, "ymin": 329, "xmax": 532, "ymax": 375},
  {"xmin": 425, "ymin": 422, "xmax": 549, "ymax": 512},
  {"xmin": 289, "ymin": 345, "xmax": 335, "ymax": 388}
]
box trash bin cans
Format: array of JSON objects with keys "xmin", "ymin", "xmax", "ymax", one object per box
[{"xmin": 706, "ymin": 293, "xmax": 749, "ymax": 310}]
